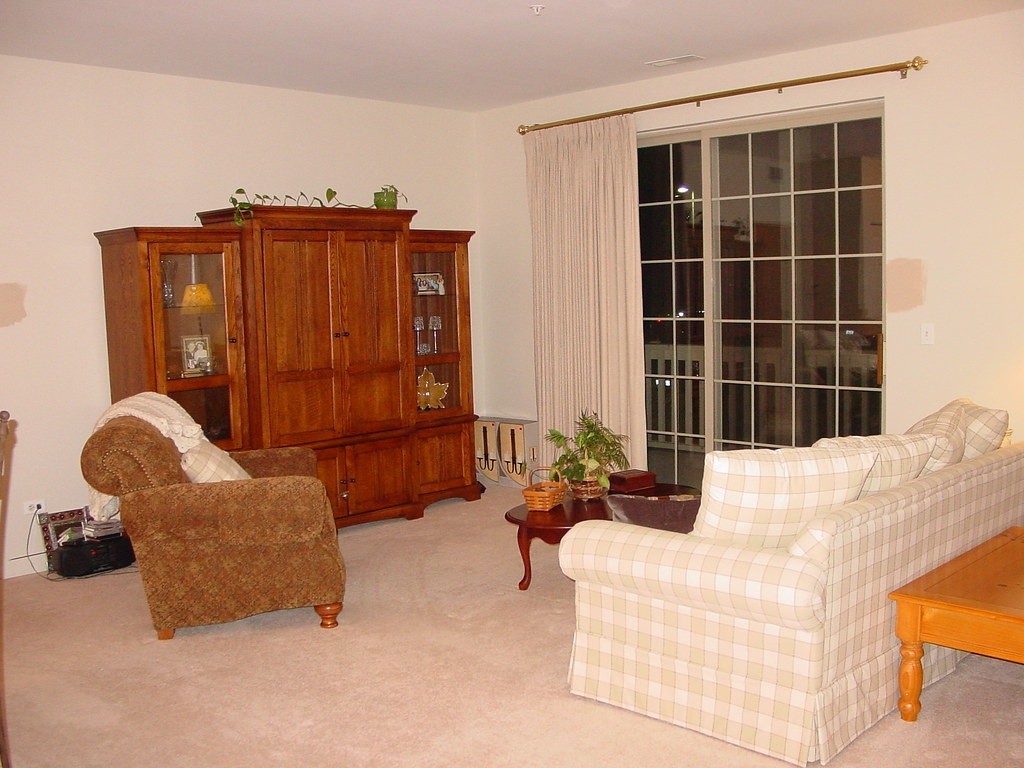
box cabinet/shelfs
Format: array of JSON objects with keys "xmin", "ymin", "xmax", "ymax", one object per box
[{"xmin": 91, "ymin": 205, "xmax": 484, "ymax": 536}]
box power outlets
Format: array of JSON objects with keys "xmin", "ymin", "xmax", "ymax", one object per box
[{"xmin": 24, "ymin": 501, "xmax": 45, "ymax": 515}]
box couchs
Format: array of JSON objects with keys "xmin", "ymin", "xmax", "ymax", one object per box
[
  {"xmin": 79, "ymin": 391, "xmax": 346, "ymax": 641},
  {"xmin": 566, "ymin": 399, "xmax": 1024, "ymax": 768}
]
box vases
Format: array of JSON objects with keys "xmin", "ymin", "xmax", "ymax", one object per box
[{"xmin": 161, "ymin": 258, "xmax": 175, "ymax": 307}]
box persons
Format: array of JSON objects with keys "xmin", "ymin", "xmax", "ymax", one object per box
[{"xmin": 186, "ymin": 341, "xmax": 208, "ymax": 369}]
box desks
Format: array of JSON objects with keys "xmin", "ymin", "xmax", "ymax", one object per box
[{"xmin": 888, "ymin": 525, "xmax": 1024, "ymax": 722}]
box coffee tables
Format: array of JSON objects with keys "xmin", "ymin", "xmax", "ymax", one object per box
[{"xmin": 504, "ymin": 481, "xmax": 700, "ymax": 592}]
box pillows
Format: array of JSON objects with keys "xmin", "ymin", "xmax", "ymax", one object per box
[
  {"xmin": 901, "ymin": 402, "xmax": 967, "ymax": 478},
  {"xmin": 809, "ymin": 433, "xmax": 937, "ymax": 501},
  {"xmin": 178, "ymin": 439, "xmax": 252, "ymax": 485},
  {"xmin": 957, "ymin": 400, "xmax": 1011, "ymax": 463},
  {"xmin": 688, "ymin": 446, "xmax": 879, "ymax": 553}
]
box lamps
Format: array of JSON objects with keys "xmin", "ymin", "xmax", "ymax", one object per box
[
  {"xmin": 428, "ymin": 315, "xmax": 443, "ymax": 354},
  {"xmin": 180, "ymin": 285, "xmax": 216, "ymax": 337},
  {"xmin": 413, "ymin": 316, "xmax": 426, "ymax": 356}
]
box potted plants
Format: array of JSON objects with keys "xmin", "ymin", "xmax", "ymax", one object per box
[
  {"xmin": 543, "ymin": 406, "xmax": 630, "ymax": 500},
  {"xmin": 228, "ymin": 184, "xmax": 408, "ymax": 226}
]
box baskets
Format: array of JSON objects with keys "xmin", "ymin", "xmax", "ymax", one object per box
[{"xmin": 522, "ymin": 468, "xmax": 567, "ymax": 511}]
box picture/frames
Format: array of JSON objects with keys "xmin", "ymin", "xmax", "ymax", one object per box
[
  {"xmin": 180, "ymin": 335, "xmax": 214, "ymax": 378},
  {"xmin": 411, "ymin": 272, "xmax": 442, "ymax": 295}
]
